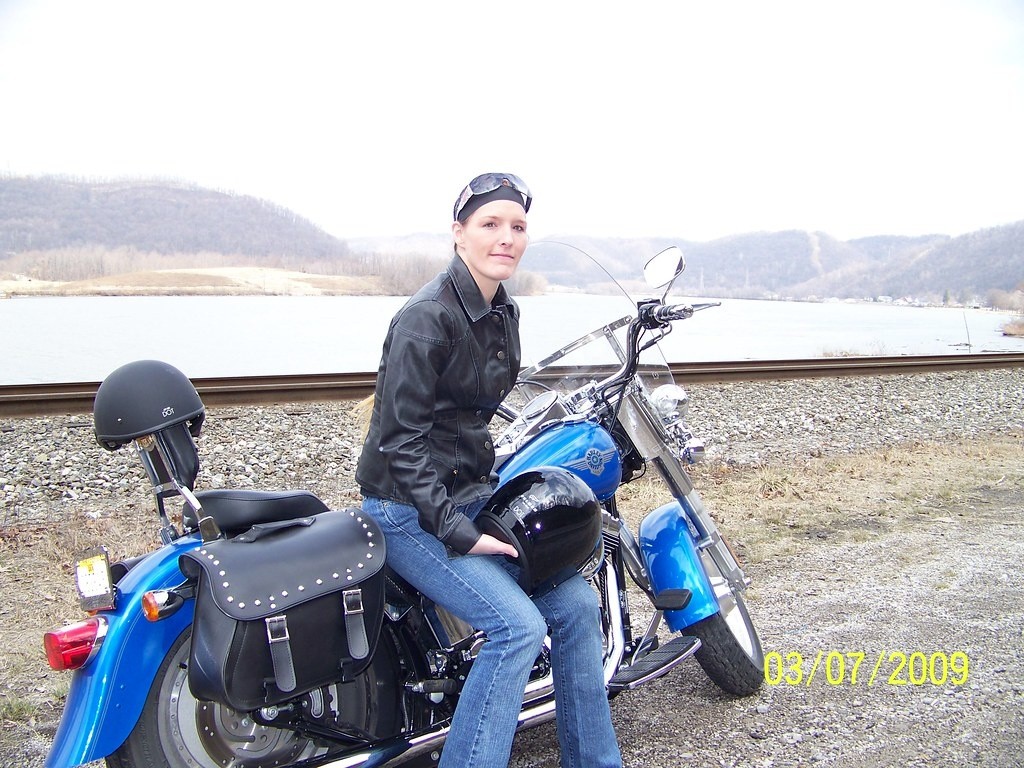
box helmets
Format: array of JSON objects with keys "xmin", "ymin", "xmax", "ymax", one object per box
[
  {"xmin": 91, "ymin": 360, "xmax": 207, "ymax": 453},
  {"xmin": 473, "ymin": 466, "xmax": 606, "ymax": 597}
]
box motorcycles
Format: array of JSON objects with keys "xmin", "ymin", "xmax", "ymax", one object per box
[{"xmin": 42, "ymin": 239, "xmax": 765, "ymax": 768}]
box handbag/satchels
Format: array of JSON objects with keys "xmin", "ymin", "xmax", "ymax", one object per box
[
  {"xmin": 110, "ymin": 549, "xmax": 150, "ymax": 586},
  {"xmin": 177, "ymin": 506, "xmax": 388, "ymax": 712}
]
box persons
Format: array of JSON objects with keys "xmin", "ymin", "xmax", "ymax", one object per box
[{"xmin": 356, "ymin": 172, "xmax": 623, "ymax": 768}]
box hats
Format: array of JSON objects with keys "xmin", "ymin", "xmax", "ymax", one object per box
[{"xmin": 457, "ymin": 187, "xmax": 527, "ymax": 220}]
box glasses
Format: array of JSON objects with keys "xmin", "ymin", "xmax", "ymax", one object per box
[{"xmin": 454, "ymin": 172, "xmax": 532, "ymax": 221}]
